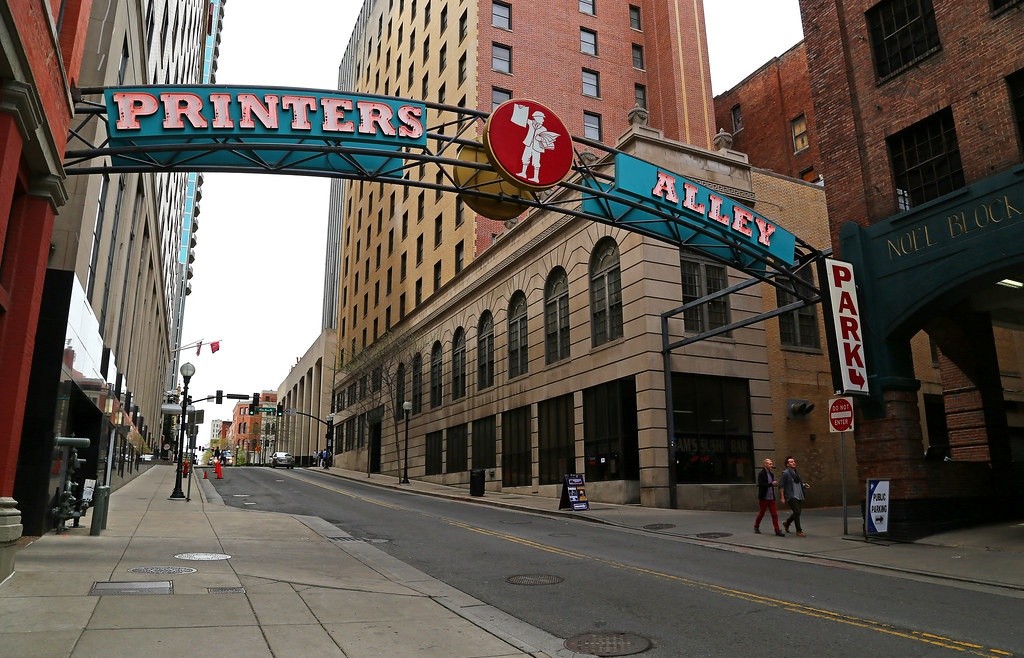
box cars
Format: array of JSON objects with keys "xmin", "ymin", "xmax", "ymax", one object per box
[
  {"xmin": 208, "ymin": 451, "xmax": 233, "ymax": 466},
  {"xmin": 269, "ymin": 452, "xmax": 295, "ymax": 469}
]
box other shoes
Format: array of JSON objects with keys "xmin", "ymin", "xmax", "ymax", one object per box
[
  {"xmin": 783, "ymin": 522, "xmax": 789, "ymax": 532},
  {"xmin": 776, "ymin": 532, "xmax": 784, "ymax": 537},
  {"xmin": 754, "ymin": 528, "xmax": 760, "ymax": 534},
  {"xmin": 797, "ymin": 532, "xmax": 804, "ymax": 537}
]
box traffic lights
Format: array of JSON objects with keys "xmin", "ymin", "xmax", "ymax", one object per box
[
  {"xmin": 277, "ymin": 405, "xmax": 283, "ymax": 417},
  {"xmin": 249, "ymin": 404, "xmax": 254, "ymax": 415}
]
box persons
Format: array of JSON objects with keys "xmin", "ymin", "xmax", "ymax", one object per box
[
  {"xmin": 312, "ymin": 448, "xmax": 332, "ymax": 467},
  {"xmin": 215, "ymin": 446, "xmax": 227, "ymax": 466},
  {"xmin": 753, "ymin": 458, "xmax": 785, "ymax": 537},
  {"xmin": 778, "ymin": 456, "xmax": 809, "ymax": 537}
]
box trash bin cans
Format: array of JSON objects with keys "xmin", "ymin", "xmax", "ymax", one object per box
[{"xmin": 468, "ymin": 469, "xmax": 486, "ymax": 496}]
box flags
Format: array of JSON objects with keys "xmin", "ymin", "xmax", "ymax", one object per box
[
  {"xmin": 196, "ymin": 342, "xmax": 202, "ymax": 356},
  {"xmin": 211, "ymin": 342, "xmax": 219, "ymax": 353}
]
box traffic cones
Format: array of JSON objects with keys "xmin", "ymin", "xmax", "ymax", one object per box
[
  {"xmin": 212, "ymin": 462, "xmax": 219, "ymax": 473},
  {"xmin": 214, "ymin": 465, "xmax": 224, "ymax": 479},
  {"xmin": 202, "ymin": 471, "xmax": 209, "ymax": 479}
]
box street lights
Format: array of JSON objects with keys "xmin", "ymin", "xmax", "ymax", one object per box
[
  {"xmin": 402, "ymin": 402, "xmax": 412, "ymax": 484},
  {"xmin": 324, "ymin": 415, "xmax": 332, "ymax": 470},
  {"xmin": 235, "ymin": 445, "xmax": 239, "ymax": 466},
  {"xmin": 170, "ymin": 362, "xmax": 195, "ymax": 498}
]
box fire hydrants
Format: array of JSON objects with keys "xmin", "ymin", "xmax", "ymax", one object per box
[{"xmin": 183, "ymin": 460, "xmax": 189, "ymax": 478}]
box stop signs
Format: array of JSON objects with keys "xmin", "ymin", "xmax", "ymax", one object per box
[{"xmin": 829, "ymin": 399, "xmax": 852, "ymax": 431}]
